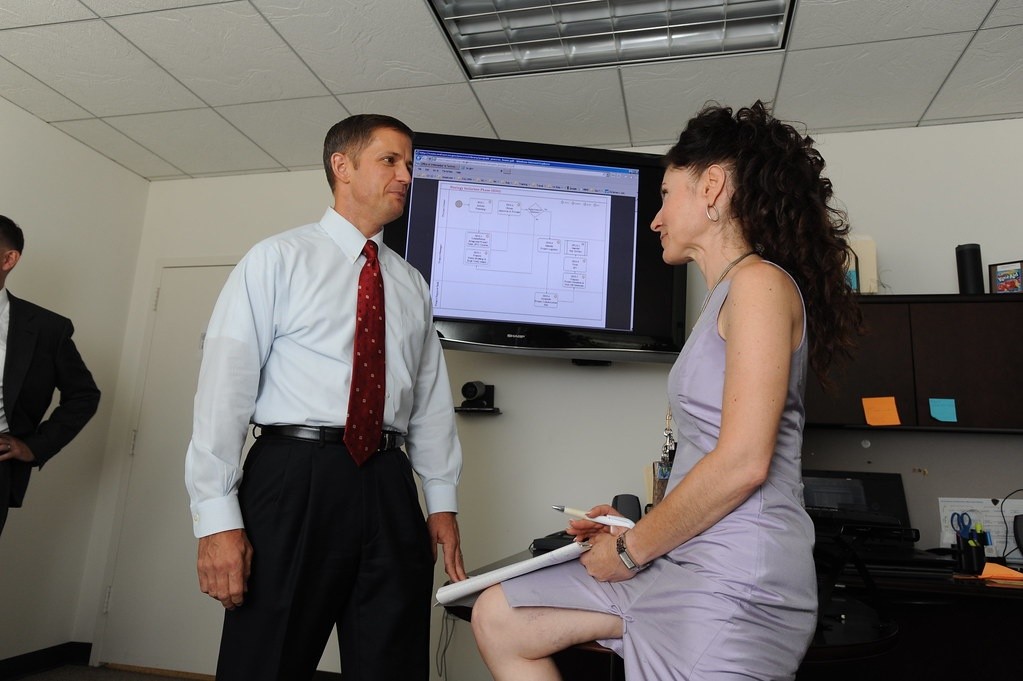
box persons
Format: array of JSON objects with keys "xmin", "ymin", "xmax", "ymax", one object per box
[
  {"xmin": 0, "ymin": 209, "xmax": 102, "ymax": 537},
  {"xmin": 470, "ymin": 101, "xmax": 869, "ymax": 681},
  {"xmin": 182, "ymin": 113, "xmax": 470, "ymax": 681}
]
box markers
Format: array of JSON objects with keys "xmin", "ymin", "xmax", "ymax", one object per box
[{"xmin": 967, "ymin": 521, "xmax": 983, "ymax": 546}]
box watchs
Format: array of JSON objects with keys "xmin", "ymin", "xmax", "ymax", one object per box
[{"xmin": 615, "ymin": 528, "xmax": 653, "ymax": 573}]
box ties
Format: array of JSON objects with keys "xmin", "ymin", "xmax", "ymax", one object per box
[{"xmin": 342, "ymin": 239, "xmax": 386, "ymax": 468}]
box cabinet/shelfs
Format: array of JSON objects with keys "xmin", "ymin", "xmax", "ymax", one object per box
[{"xmin": 804, "ymin": 293, "xmax": 1023, "ymax": 437}]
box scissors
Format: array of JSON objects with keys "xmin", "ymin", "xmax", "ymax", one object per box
[{"xmin": 950, "ymin": 512, "xmax": 971, "ymax": 539}]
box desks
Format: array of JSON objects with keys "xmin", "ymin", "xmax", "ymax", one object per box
[{"xmin": 443, "ymin": 547, "xmax": 1023, "ymax": 681}]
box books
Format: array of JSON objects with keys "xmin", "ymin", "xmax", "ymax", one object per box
[{"xmin": 435, "ymin": 540, "xmax": 593, "ymax": 605}]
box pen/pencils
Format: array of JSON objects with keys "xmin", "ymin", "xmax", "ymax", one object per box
[{"xmin": 549, "ymin": 504, "xmax": 637, "ymax": 528}]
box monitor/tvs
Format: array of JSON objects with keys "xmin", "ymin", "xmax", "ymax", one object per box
[{"xmin": 382, "ymin": 132, "xmax": 687, "ymax": 353}]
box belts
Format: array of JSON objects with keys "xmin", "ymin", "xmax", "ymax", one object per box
[{"xmin": 261, "ymin": 425, "xmax": 406, "ymax": 451}]
